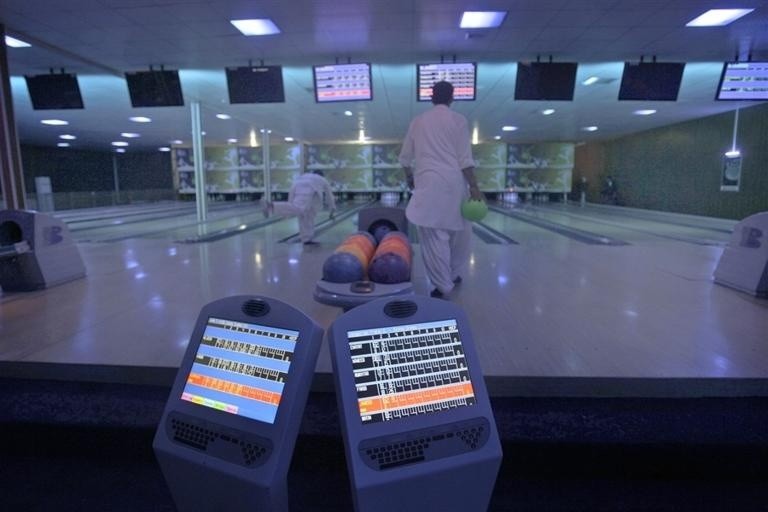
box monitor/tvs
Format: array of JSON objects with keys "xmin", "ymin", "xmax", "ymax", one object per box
[
  {"xmin": 175, "ymin": 309, "xmax": 301, "ymax": 428},
  {"xmin": 345, "ymin": 314, "xmax": 481, "ymax": 430},
  {"xmin": 123, "ymin": 70, "xmax": 186, "ymax": 111},
  {"xmin": 513, "ymin": 58, "xmax": 578, "ymax": 103},
  {"xmin": 224, "ymin": 66, "xmax": 288, "ymax": 105},
  {"xmin": 712, "ymin": 62, "xmax": 767, "ymax": 103},
  {"xmin": 617, "ymin": 61, "xmax": 688, "ymax": 103},
  {"xmin": 20, "ymin": 72, "xmax": 86, "ymax": 113},
  {"xmin": 310, "ymin": 61, "xmax": 374, "ymax": 103},
  {"xmin": 415, "ymin": 62, "xmax": 478, "ymax": 102}
]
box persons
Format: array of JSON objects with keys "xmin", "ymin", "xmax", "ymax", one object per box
[
  {"xmin": 600, "ymin": 176, "xmax": 615, "ymax": 195},
  {"xmin": 260, "ymin": 170, "xmax": 336, "ymax": 244},
  {"xmin": 398, "ymin": 81, "xmax": 487, "ymax": 300}
]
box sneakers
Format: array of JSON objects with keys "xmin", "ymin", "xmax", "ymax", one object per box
[
  {"xmin": 453, "ymin": 277, "xmax": 463, "ymax": 284},
  {"xmin": 304, "ymin": 241, "xmax": 318, "ymax": 246},
  {"xmin": 431, "ymin": 287, "xmax": 443, "ymax": 298},
  {"xmin": 261, "ymin": 196, "xmax": 270, "ymax": 217}
]
